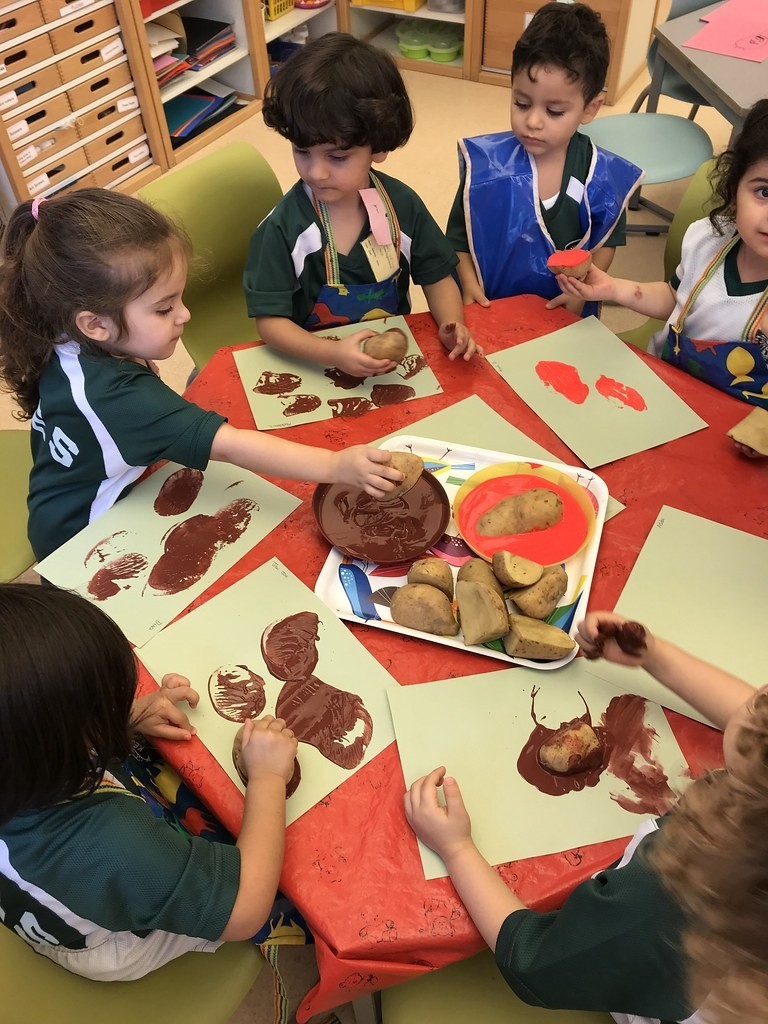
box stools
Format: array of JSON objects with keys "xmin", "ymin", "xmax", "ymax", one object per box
[{"xmin": 577, "ymin": 114, "xmax": 713, "ymax": 186}]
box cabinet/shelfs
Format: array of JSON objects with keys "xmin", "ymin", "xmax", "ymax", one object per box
[{"xmin": 0, "ymin": 0, "xmax": 673, "ymax": 223}]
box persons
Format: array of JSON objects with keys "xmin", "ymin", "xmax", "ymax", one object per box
[
  {"xmin": 242, "ymin": 31, "xmax": 485, "ymax": 377},
  {"xmin": 0, "ymin": 187, "xmax": 406, "ymax": 564},
  {"xmin": 554, "ymin": 97, "xmax": 768, "ymax": 458},
  {"xmin": 0, "ymin": 581, "xmax": 298, "ymax": 983},
  {"xmin": 404, "ymin": 609, "xmax": 768, "ymax": 1024},
  {"xmin": 446, "ymin": 3, "xmax": 645, "ymax": 320}
]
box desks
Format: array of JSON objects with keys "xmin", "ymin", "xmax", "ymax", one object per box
[
  {"xmin": 133, "ymin": 295, "xmax": 768, "ymax": 957},
  {"xmin": 646, "ymin": 0, "xmax": 768, "ymax": 157}
]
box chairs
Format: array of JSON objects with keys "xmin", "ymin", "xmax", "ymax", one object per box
[{"xmin": 629, "ymin": 0, "xmax": 721, "ymax": 121}]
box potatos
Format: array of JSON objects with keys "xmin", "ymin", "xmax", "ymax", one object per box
[
  {"xmin": 233, "ymin": 724, "xmax": 294, "ymax": 786},
  {"xmin": 387, "ymin": 550, "xmax": 577, "ymax": 660},
  {"xmin": 537, "ymin": 722, "xmax": 601, "ymax": 777},
  {"xmin": 547, "ymin": 250, "xmax": 592, "ymax": 279},
  {"xmin": 475, "ymin": 489, "xmax": 563, "ymax": 536},
  {"xmin": 364, "ymin": 331, "xmax": 406, "ymax": 363},
  {"xmin": 376, "ymin": 451, "xmax": 424, "ymax": 501}
]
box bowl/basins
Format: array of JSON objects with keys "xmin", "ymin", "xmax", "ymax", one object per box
[
  {"xmin": 312, "ymin": 469, "xmax": 451, "ymax": 563},
  {"xmin": 453, "ymin": 462, "xmax": 595, "ymax": 567}
]
